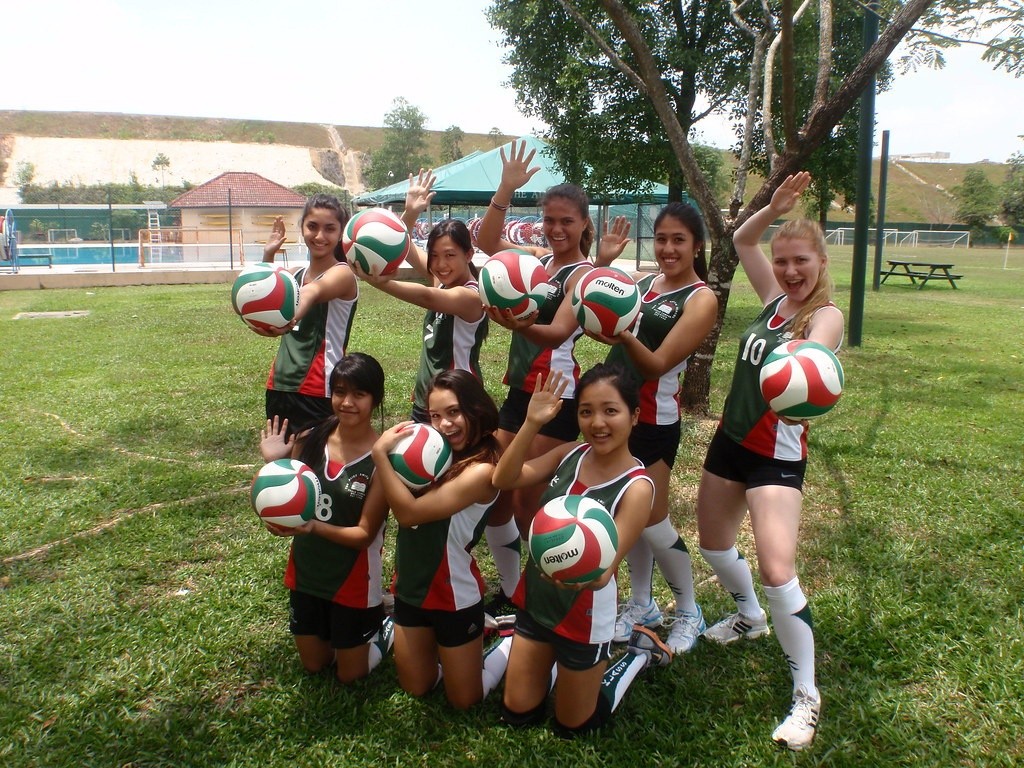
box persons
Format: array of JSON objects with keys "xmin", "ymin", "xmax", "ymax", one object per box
[
  {"xmin": 346, "ymin": 169, "xmax": 489, "ymax": 423},
  {"xmin": 698, "ymin": 171, "xmax": 846, "ymax": 751},
  {"xmin": 264, "ymin": 195, "xmax": 359, "ymax": 449},
  {"xmin": 258, "ymin": 354, "xmax": 395, "ymax": 682},
  {"xmin": 492, "ymin": 365, "xmax": 672, "ymax": 731},
  {"xmin": 477, "ymin": 141, "xmax": 595, "ymax": 621},
  {"xmin": 585, "ymin": 202, "xmax": 718, "ymax": 657},
  {"xmin": 374, "ymin": 369, "xmax": 516, "ymax": 708}
]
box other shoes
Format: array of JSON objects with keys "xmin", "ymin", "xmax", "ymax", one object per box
[
  {"xmin": 381, "ymin": 594, "xmax": 395, "ymax": 614},
  {"xmin": 482, "ymin": 612, "xmax": 499, "ymax": 637},
  {"xmin": 495, "ymin": 614, "xmax": 517, "ymax": 637}
]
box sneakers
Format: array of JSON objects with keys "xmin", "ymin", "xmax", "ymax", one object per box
[
  {"xmin": 611, "ymin": 598, "xmax": 663, "ymax": 643},
  {"xmin": 626, "ymin": 626, "xmax": 672, "ymax": 666},
  {"xmin": 771, "ymin": 684, "xmax": 822, "ymax": 751},
  {"xmin": 699, "ymin": 608, "xmax": 771, "ymax": 645},
  {"xmin": 482, "ymin": 586, "xmax": 517, "ymax": 624},
  {"xmin": 661, "ymin": 602, "xmax": 707, "ymax": 653}
]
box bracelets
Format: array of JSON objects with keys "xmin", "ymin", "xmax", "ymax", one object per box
[{"xmin": 491, "ymin": 197, "xmax": 510, "ymax": 210}]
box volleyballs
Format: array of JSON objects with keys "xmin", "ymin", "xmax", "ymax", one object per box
[
  {"xmin": 250, "ymin": 458, "xmax": 322, "ymax": 531},
  {"xmin": 759, "ymin": 339, "xmax": 844, "ymax": 422},
  {"xmin": 231, "ymin": 262, "xmax": 299, "ymax": 329},
  {"xmin": 529, "ymin": 493, "xmax": 619, "ymax": 587},
  {"xmin": 386, "ymin": 423, "xmax": 453, "ymax": 494},
  {"xmin": 342, "ymin": 207, "xmax": 410, "ymax": 276},
  {"xmin": 478, "ymin": 248, "xmax": 547, "ymax": 321},
  {"xmin": 572, "ymin": 266, "xmax": 642, "ymax": 338}
]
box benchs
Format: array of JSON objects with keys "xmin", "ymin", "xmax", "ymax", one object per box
[
  {"xmin": 18, "ymin": 254, "xmax": 53, "ymax": 268},
  {"xmin": 910, "ymin": 271, "xmax": 964, "ymax": 277},
  {"xmin": 881, "ymin": 271, "xmax": 927, "ymax": 277}
]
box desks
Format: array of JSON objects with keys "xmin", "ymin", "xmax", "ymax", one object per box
[{"xmin": 880, "ymin": 260, "xmax": 961, "ymax": 290}]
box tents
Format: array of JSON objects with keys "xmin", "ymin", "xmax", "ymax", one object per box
[{"xmin": 350, "ymin": 135, "xmax": 689, "ymax": 259}]
